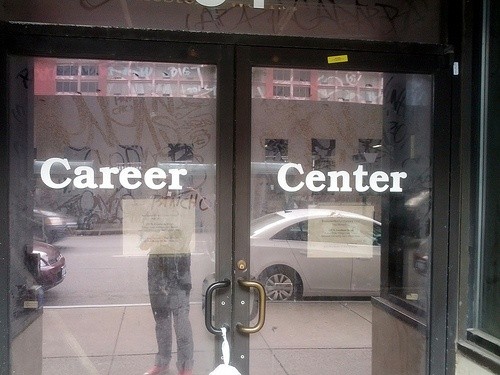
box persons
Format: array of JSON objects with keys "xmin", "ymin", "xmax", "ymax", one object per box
[{"xmin": 138, "ymin": 183, "xmax": 194, "ymax": 375}]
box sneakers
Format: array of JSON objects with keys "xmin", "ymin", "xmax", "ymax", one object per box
[
  {"xmin": 177, "ymin": 369, "xmax": 192, "ymax": 375},
  {"xmin": 144, "ymin": 364, "xmax": 169, "ymax": 375}
]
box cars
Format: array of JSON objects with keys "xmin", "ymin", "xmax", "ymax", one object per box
[
  {"xmin": 33, "ymin": 207, "xmax": 79, "ymax": 241},
  {"xmin": 250, "ymin": 208, "xmax": 427, "ymax": 303},
  {"xmin": 31, "ymin": 240, "xmax": 66, "ymax": 294}
]
